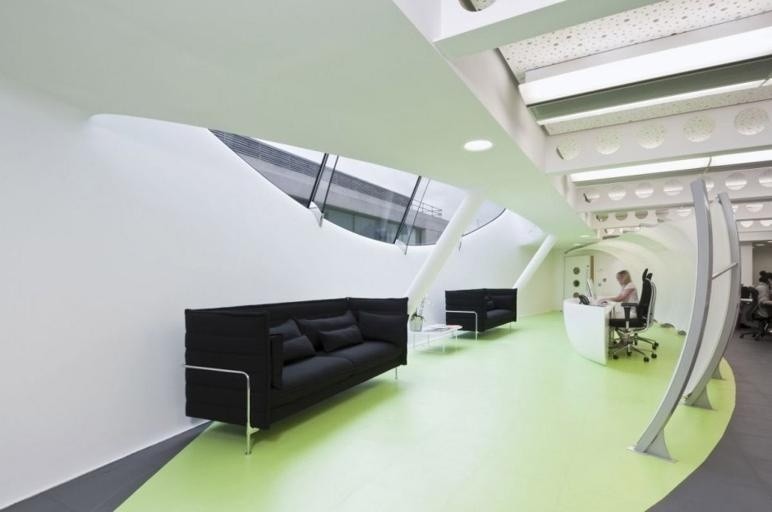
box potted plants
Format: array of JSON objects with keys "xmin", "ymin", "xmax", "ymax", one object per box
[{"xmin": 410, "ymin": 298, "xmax": 430, "ymax": 332}]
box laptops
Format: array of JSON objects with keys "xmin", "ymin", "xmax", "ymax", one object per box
[{"xmin": 587, "ymin": 279, "xmax": 597, "ymax": 298}]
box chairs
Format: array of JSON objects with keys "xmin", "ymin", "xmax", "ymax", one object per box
[
  {"xmin": 613, "ymin": 273, "xmax": 659, "ymax": 350},
  {"xmin": 609, "ymin": 268, "xmax": 657, "ymax": 362},
  {"xmin": 739, "ymin": 286, "xmax": 771, "ymax": 341}
]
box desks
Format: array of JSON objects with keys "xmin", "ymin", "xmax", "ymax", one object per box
[
  {"xmin": 563, "ymin": 295, "xmax": 617, "ymax": 365},
  {"xmin": 408, "ymin": 323, "xmax": 464, "ymax": 354},
  {"xmin": 739, "ymin": 297, "xmax": 771, "ymax": 308}
]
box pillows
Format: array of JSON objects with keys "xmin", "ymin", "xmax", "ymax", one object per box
[{"xmin": 268, "ymin": 309, "xmax": 406, "ymax": 390}]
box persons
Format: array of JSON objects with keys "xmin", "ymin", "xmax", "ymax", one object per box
[
  {"xmin": 759, "ymin": 270, "xmax": 771, "ymax": 280},
  {"xmin": 596, "ymin": 270, "xmax": 640, "ymax": 344},
  {"xmin": 754, "ymin": 276, "xmax": 770, "ymax": 333}
]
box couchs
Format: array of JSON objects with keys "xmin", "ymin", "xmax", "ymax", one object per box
[
  {"xmin": 183, "ymin": 296, "xmax": 409, "ymax": 455},
  {"xmin": 445, "ymin": 288, "xmax": 517, "ymax": 340}
]
